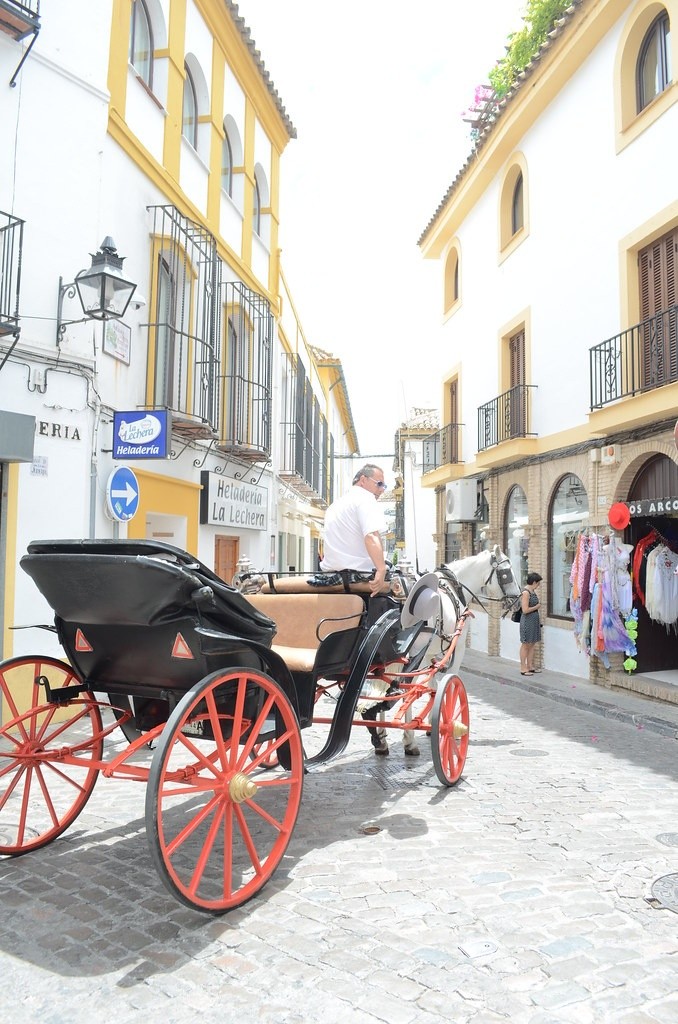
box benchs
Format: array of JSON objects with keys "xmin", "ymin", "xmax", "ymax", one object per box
[
  {"xmin": 259, "ymin": 575, "xmax": 392, "ymax": 595},
  {"xmin": 242, "ymin": 593, "xmax": 367, "ymax": 673}
]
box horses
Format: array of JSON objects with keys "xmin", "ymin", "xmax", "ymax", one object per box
[{"xmin": 368, "ymin": 545, "xmax": 523, "ymax": 756}]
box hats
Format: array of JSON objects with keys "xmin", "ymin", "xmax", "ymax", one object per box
[
  {"xmin": 608, "ymin": 503, "xmax": 630, "ymax": 530},
  {"xmin": 401, "ymin": 573, "xmax": 439, "ymax": 629}
]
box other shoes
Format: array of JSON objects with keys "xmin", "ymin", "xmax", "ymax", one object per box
[
  {"xmin": 521, "ymin": 671, "xmax": 533, "ymax": 676},
  {"xmin": 529, "ymin": 668, "xmax": 541, "ymax": 673}
]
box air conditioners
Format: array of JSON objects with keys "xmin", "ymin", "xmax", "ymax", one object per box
[{"xmin": 445, "ymin": 479, "xmax": 478, "ymax": 524}]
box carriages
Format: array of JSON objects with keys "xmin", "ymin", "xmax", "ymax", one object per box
[{"xmin": 0, "ymin": 538, "xmax": 522, "ymax": 916}]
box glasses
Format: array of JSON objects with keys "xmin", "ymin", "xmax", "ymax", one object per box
[{"xmin": 365, "ymin": 476, "xmax": 387, "ymax": 490}]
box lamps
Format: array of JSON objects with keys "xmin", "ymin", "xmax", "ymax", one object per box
[{"xmin": 56, "ymin": 235, "xmax": 137, "ymax": 347}]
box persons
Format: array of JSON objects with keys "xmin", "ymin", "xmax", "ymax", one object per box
[
  {"xmin": 319, "ymin": 463, "xmax": 387, "ymax": 598},
  {"xmin": 519, "ymin": 572, "xmax": 543, "ymax": 676}
]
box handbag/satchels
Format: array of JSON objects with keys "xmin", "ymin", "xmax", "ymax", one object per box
[{"xmin": 511, "ymin": 606, "xmax": 521, "ymax": 622}]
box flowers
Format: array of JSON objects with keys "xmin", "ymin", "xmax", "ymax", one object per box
[{"xmin": 461, "ymin": 86, "xmax": 505, "ymax": 120}]
